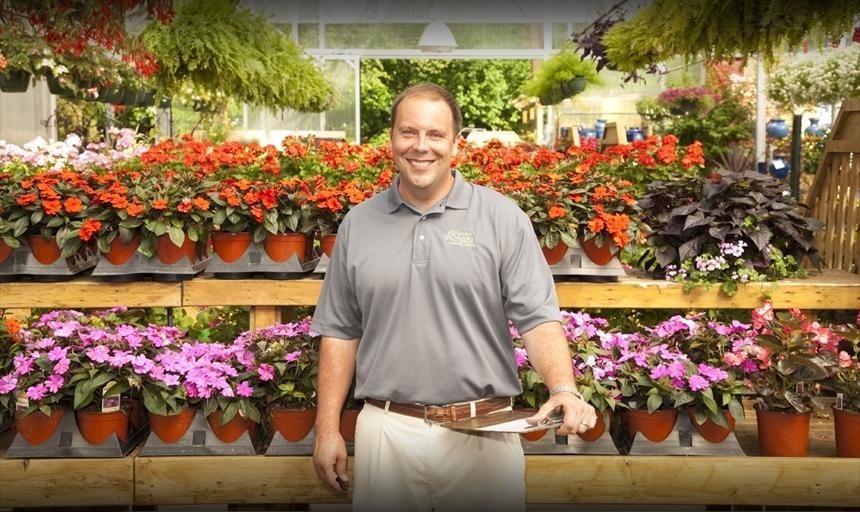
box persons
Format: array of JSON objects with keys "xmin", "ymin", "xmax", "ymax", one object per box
[{"xmin": 309, "ymin": 84, "xmax": 598, "ymax": 512}]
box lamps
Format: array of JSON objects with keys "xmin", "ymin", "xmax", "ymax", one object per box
[{"xmin": 417, "ymin": 0, "xmax": 457, "ymax": 54}]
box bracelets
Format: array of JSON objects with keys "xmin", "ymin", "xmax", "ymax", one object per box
[{"xmin": 550, "ymin": 384, "xmax": 585, "ymax": 400}]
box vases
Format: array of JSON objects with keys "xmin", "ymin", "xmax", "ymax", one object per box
[
  {"xmin": 30, "ymin": 234, "xmax": 69, "ymax": 281},
  {"xmin": 64, "ymin": 238, "xmax": 99, "ymax": 279},
  {"xmin": 99, "ymin": 233, "xmax": 146, "ymax": 282},
  {"xmin": 0, "ymin": 234, "xmax": 23, "ymax": 281},
  {"xmin": 316, "ymin": 233, "xmax": 336, "ymax": 281},
  {"xmin": 517, "ymin": 407, "xmax": 558, "ymax": 441},
  {"xmin": 10, "ymin": 400, "xmax": 65, "ymax": 445},
  {"xmin": 263, "ymin": 404, "xmax": 316, "ymax": 441},
  {"xmin": 538, "ymin": 240, "xmax": 570, "ymax": 281},
  {"xmin": 753, "ymin": 402, "xmax": 815, "ymax": 459},
  {"xmin": 767, "ymin": 117, "xmax": 787, "ymax": 139},
  {"xmin": 143, "ymin": 404, "xmax": 199, "ymax": 447},
  {"xmin": 564, "ymin": 409, "xmax": 612, "ymax": 443},
  {"xmin": 806, "ymin": 117, "xmax": 823, "ymax": 137},
  {"xmin": 621, "ymin": 410, "xmax": 679, "ymax": 448},
  {"xmin": 579, "ymin": 234, "xmax": 616, "ymax": 281},
  {"xmin": 154, "ymin": 232, "xmax": 197, "ymax": 282},
  {"xmin": 210, "ymin": 232, "xmax": 253, "ymax": 279},
  {"xmin": 337, "ymin": 407, "xmax": 363, "ymax": 444},
  {"xmin": 771, "ymin": 155, "xmax": 788, "ymax": 177},
  {"xmin": 687, "ymin": 406, "xmax": 736, "ymax": 444},
  {"xmin": 203, "ymin": 407, "xmax": 257, "ymax": 449},
  {"xmin": 833, "ymin": 409, "xmax": 859, "ymax": 457},
  {"xmin": 77, "ymin": 401, "xmax": 131, "ymax": 452},
  {"xmin": 265, "ymin": 233, "xmax": 307, "ymax": 279}
]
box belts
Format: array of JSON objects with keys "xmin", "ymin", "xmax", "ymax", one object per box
[{"xmin": 365, "ymin": 395, "xmax": 510, "ymax": 424}]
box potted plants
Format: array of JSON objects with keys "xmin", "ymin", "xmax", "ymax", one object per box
[{"xmin": 520, "ymin": 50, "xmax": 606, "ymax": 107}]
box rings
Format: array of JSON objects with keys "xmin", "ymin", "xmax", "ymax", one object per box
[{"xmin": 581, "ymin": 422, "xmax": 588, "ymax": 430}]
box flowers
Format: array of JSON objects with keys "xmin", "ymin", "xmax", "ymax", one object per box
[
  {"xmin": 1, "ymin": 32, "xmax": 173, "ymax": 109},
  {"xmin": 1, "ymin": 306, "xmax": 860, "ymax": 410},
  {"xmin": 647, "ymin": 171, "xmax": 828, "ymax": 297},
  {"xmin": 0, "ymin": 120, "xmax": 704, "ymax": 237}
]
